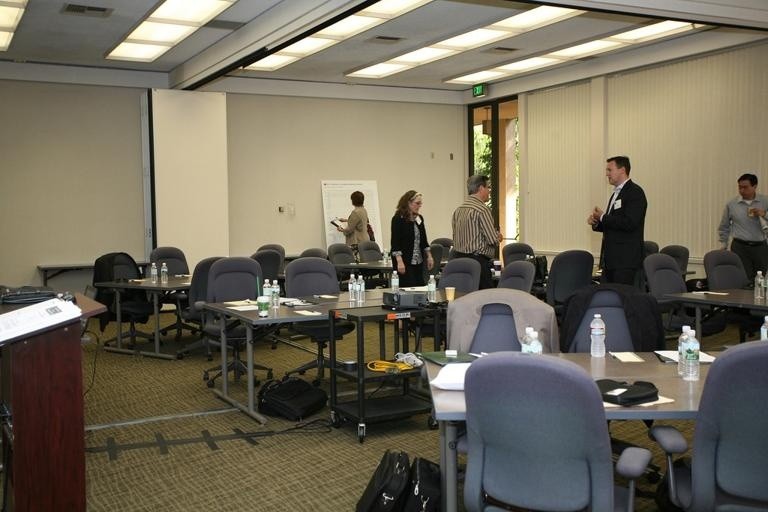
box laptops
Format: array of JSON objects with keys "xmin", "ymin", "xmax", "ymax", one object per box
[{"xmin": 422, "ymin": 350, "xmax": 479, "ymax": 367}]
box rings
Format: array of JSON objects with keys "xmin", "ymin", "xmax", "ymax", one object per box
[{"xmin": 591, "ymin": 217, "xmax": 593, "ymax": 219}]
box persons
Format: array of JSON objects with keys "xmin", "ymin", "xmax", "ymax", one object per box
[
  {"xmin": 718, "ymin": 173, "xmax": 768, "ymax": 284},
  {"xmin": 329, "ymin": 191, "xmax": 376, "ymax": 248},
  {"xmin": 587, "ymin": 155, "xmax": 647, "ymax": 285},
  {"xmin": 451, "ymin": 174, "xmax": 504, "ymax": 281},
  {"xmin": 390, "ymin": 190, "xmax": 434, "ymax": 287}
]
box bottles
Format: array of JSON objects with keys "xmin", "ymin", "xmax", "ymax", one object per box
[
  {"xmin": 448, "ymin": 245, "xmax": 454, "ymax": 260},
  {"xmin": 383, "ymin": 249, "xmax": 389, "ymax": 265},
  {"xmin": 532, "ymin": 329, "xmax": 543, "ymax": 355},
  {"xmin": 160, "ymin": 262, "xmax": 168, "ymax": 285},
  {"xmin": 676, "ymin": 324, "xmax": 691, "ymax": 375},
  {"xmin": 490, "ymin": 269, "xmax": 495, "ymax": 280},
  {"xmin": 524, "ymin": 254, "xmax": 529, "ymax": 262},
  {"xmin": 355, "ymin": 275, "xmax": 365, "ymax": 302},
  {"xmin": 544, "ymin": 276, "xmax": 548, "ymax": 280},
  {"xmin": 151, "ymin": 263, "xmax": 158, "ymax": 284},
  {"xmin": 681, "ymin": 329, "xmax": 699, "ymax": 382},
  {"xmin": 348, "ymin": 273, "xmax": 357, "ymax": 300},
  {"xmin": 520, "ymin": 325, "xmax": 535, "ymax": 354},
  {"xmin": 390, "ymin": 271, "xmax": 399, "ymax": 292},
  {"xmin": 528, "ymin": 255, "xmax": 533, "ymax": 259},
  {"xmin": 753, "ymin": 269, "xmax": 768, "ymax": 301},
  {"xmin": 427, "ymin": 274, "xmax": 436, "ymax": 303},
  {"xmin": 262, "ymin": 279, "xmax": 272, "ymax": 307},
  {"xmin": 590, "ymin": 312, "xmax": 607, "ymax": 360},
  {"xmin": 759, "ymin": 316, "xmax": 767, "ymax": 341},
  {"xmin": 271, "ymin": 279, "xmax": 280, "ymax": 308}
]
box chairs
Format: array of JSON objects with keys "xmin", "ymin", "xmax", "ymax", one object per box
[
  {"xmin": 199, "ymin": 255, "xmax": 466, "ymax": 426},
  {"xmin": 446, "ymin": 287, "xmax": 556, "ymax": 454},
  {"xmin": 563, "ymin": 283, "xmax": 663, "ymax": 352},
  {"xmin": 654, "ymin": 337, "xmax": 768, "ymax": 512},
  {"xmin": 642, "ymin": 238, "xmax": 767, "ymax": 345},
  {"xmin": 458, "ymin": 351, "xmax": 652, "ymax": 512},
  {"xmin": 93, "ymin": 237, "xmax": 596, "ymax": 361}
]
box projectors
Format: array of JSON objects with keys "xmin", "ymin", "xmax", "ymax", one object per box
[{"xmin": 383, "ymin": 290, "xmax": 428, "ymax": 310}]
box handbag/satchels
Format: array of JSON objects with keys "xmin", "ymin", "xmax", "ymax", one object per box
[
  {"xmin": 356, "ymin": 447, "xmax": 441, "ymax": 511},
  {"xmin": 367, "ymin": 224, "xmax": 375, "ymax": 241},
  {"xmin": 258, "ymin": 378, "xmax": 328, "ymax": 421}
]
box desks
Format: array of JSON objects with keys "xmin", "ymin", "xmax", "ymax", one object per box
[
  {"xmin": 35, "ymin": 259, "xmax": 149, "ymax": 289},
  {"xmin": 422, "ymin": 349, "xmax": 728, "ymax": 512}
]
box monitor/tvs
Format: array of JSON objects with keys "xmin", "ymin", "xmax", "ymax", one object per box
[{"xmin": 80, "ymin": 285, "xmax": 99, "ymax": 330}]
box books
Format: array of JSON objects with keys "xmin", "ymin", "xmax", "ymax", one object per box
[
  {"xmin": 421, "ymin": 345, "xmax": 479, "ymax": 366},
  {"xmin": 330, "ymin": 217, "xmax": 344, "ymax": 230},
  {"xmin": 257, "ymin": 295, "xmax": 298, "ymax": 305}
]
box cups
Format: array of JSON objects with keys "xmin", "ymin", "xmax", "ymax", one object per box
[
  {"xmin": 444, "ymin": 287, "xmax": 455, "ymax": 302},
  {"xmin": 746, "ymin": 207, "xmax": 755, "ymax": 216},
  {"xmin": 494, "ymin": 271, "xmax": 501, "ymax": 277},
  {"xmin": 440, "ymin": 256, "xmax": 448, "ymax": 263},
  {"xmin": 256, "ymin": 295, "xmax": 269, "ymax": 317},
  {"xmin": 343, "ymin": 361, "xmax": 356, "ymax": 367}
]
